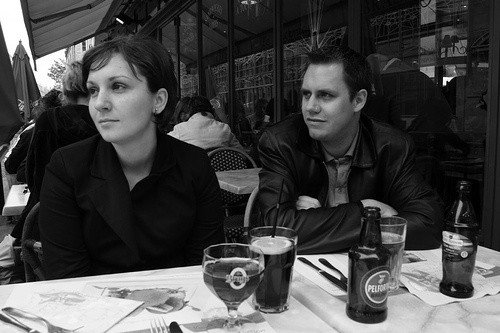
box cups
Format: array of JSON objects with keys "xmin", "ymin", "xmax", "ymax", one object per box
[
  {"xmin": 249, "ymin": 225, "xmax": 298, "ymax": 315},
  {"xmin": 360, "ymin": 216, "xmax": 408, "ymax": 295}
]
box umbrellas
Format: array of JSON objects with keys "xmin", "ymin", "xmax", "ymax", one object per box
[{"xmin": 0, "ymin": 22, "xmax": 42, "ymax": 145}]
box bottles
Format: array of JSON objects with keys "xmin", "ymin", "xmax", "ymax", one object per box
[
  {"xmin": 347, "ymin": 205, "xmax": 392, "ymax": 324},
  {"xmin": 439, "ymin": 178, "xmax": 480, "ymax": 298}
]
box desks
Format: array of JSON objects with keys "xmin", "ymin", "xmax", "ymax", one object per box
[
  {"xmin": 0, "ymin": 242, "xmax": 500, "ymax": 333},
  {"xmin": 214, "ymin": 168, "xmax": 262, "ymax": 195}
]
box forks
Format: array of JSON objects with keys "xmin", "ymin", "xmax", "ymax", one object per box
[
  {"xmin": 318, "ymin": 257, "xmax": 349, "ymax": 286},
  {"xmin": 2, "ymin": 308, "xmax": 83, "ymax": 333},
  {"xmin": 148, "ymin": 315, "xmax": 169, "ymax": 333}
]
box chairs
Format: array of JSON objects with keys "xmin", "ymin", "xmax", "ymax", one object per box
[
  {"xmin": 2, "ymin": 184, "xmax": 31, "ymax": 216},
  {"xmin": 207, "ymin": 147, "xmax": 263, "ymax": 259}
]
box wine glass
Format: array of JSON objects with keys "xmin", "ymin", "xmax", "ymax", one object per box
[{"xmin": 199, "ymin": 243, "xmax": 266, "ymax": 333}]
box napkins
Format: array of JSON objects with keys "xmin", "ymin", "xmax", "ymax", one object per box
[
  {"xmin": 292, "ymin": 253, "xmax": 353, "ymax": 296},
  {"xmin": 37, "ymin": 295, "xmax": 145, "ymax": 333}
]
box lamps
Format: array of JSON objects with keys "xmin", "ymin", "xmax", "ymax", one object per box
[{"xmin": 114, "ymin": 14, "xmax": 153, "ymax": 27}]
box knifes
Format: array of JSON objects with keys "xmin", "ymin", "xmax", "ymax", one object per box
[{"xmin": 297, "ymin": 256, "xmax": 347, "ymax": 292}]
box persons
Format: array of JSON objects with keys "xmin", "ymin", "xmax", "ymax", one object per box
[
  {"xmin": 258, "ymin": 47, "xmax": 447, "ymax": 255},
  {"xmin": 5, "ymin": 60, "xmax": 247, "ymax": 237},
  {"xmin": 219, "ymin": 51, "xmax": 462, "ymax": 202},
  {"xmin": 38, "ymin": 33, "xmax": 225, "ymax": 281}
]
